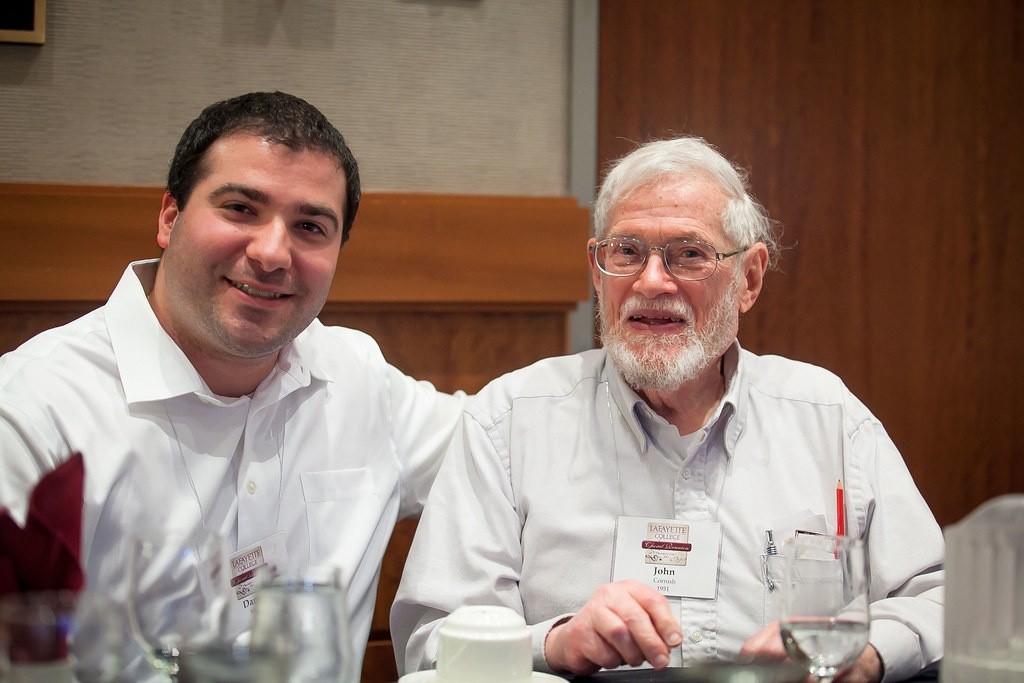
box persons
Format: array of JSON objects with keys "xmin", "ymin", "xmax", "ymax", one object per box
[
  {"xmin": 387, "ymin": 134, "xmax": 955, "ymax": 683},
  {"xmin": 1, "ymin": 90, "xmax": 466, "ymax": 683}
]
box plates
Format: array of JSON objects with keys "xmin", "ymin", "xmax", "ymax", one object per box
[{"xmin": 399, "ymin": 667, "xmax": 572, "ymax": 682}]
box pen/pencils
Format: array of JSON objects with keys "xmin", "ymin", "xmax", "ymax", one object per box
[{"xmin": 835, "ymin": 479, "xmax": 846, "ymax": 561}]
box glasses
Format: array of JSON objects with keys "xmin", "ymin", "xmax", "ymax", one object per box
[{"xmin": 587, "ymin": 237, "xmax": 751, "ymax": 279}]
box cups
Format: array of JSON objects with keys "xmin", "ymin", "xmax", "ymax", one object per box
[
  {"xmin": 437, "ymin": 604, "xmax": 534, "ymax": 682},
  {"xmin": 253, "ymin": 585, "xmax": 352, "ymax": 682},
  {"xmin": 131, "ymin": 525, "xmax": 235, "ymax": 683}
]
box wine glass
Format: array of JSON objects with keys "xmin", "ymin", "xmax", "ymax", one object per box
[{"xmin": 782, "ymin": 534, "xmax": 869, "ymax": 683}]
